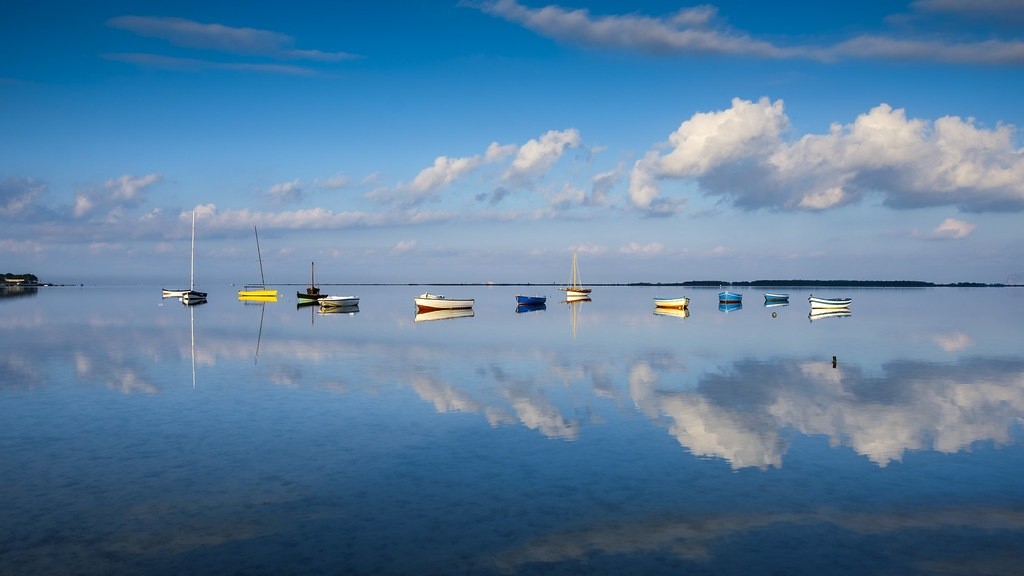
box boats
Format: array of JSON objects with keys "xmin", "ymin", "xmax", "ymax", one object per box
[
  {"xmin": 808, "ymin": 308, "xmax": 852, "ymax": 320},
  {"xmin": 808, "ymin": 294, "xmax": 852, "ymax": 309},
  {"xmin": 317, "ymin": 295, "xmax": 359, "ymax": 307},
  {"xmin": 718, "ymin": 302, "xmax": 742, "ymax": 313},
  {"xmin": 653, "ymin": 296, "xmax": 690, "ymax": 307},
  {"xmin": 653, "ymin": 306, "xmax": 691, "ymax": 318},
  {"xmin": 763, "ymin": 292, "xmax": 789, "ymax": 301},
  {"xmin": 764, "ymin": 300, "xmax": 789, "ymax": 307},
  {"xmin": 296, "ymin": 261, "xmax": 328, "ymax": 299},
  {"xmin": 514, "ymin": 295, "xmax": 547, "ymax": 305},
  {"xmin": 162, "ymin": 288, "xmax": 191, "ymax": 294},
  {"xmin": 414, "ymin": 307, "xmax": 475, "ymax": 322},
  {"xmin": 415, "ymin": 291, "xmax": 475, "ymax": 309},
  {"xmin": 718, "ymin": 290, "xmax": 742, "ymax": 302},
  {"xmin": 163, "ymin": 293, "xmax": 184, "ymax": 297},
  {"xmin": 297, "ymin": 298, "xmax": 319, "ymax": 326},
  {"xmin": 317, "ymin": 306, "xmax": 359, "ymax": 313},
  {"xmin": 515, "ymin": 304, "xmax": 547, "ymax": 313}
]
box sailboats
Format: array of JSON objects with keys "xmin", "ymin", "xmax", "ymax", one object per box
[
  {"xmin": 237, "ymin": 225, "xmax": 278, "ymax": 296},
  {"xmin": 182, "ymin": 298, "xmax": 208, "ymax": 388},
  {"xmin": 566, "ymin": 295, "xmax": 593, "ymax": 337},
  {"xmin": 237, "ymin": 295, "xmax": 278, "ymax": 366},
  {"xmin": 182, "ymin": 209, "xmax": 208, "ymax": 299},
  {"xmin": 565, "ymin": 252, "xmax": 592, "ymax": 296}
]
